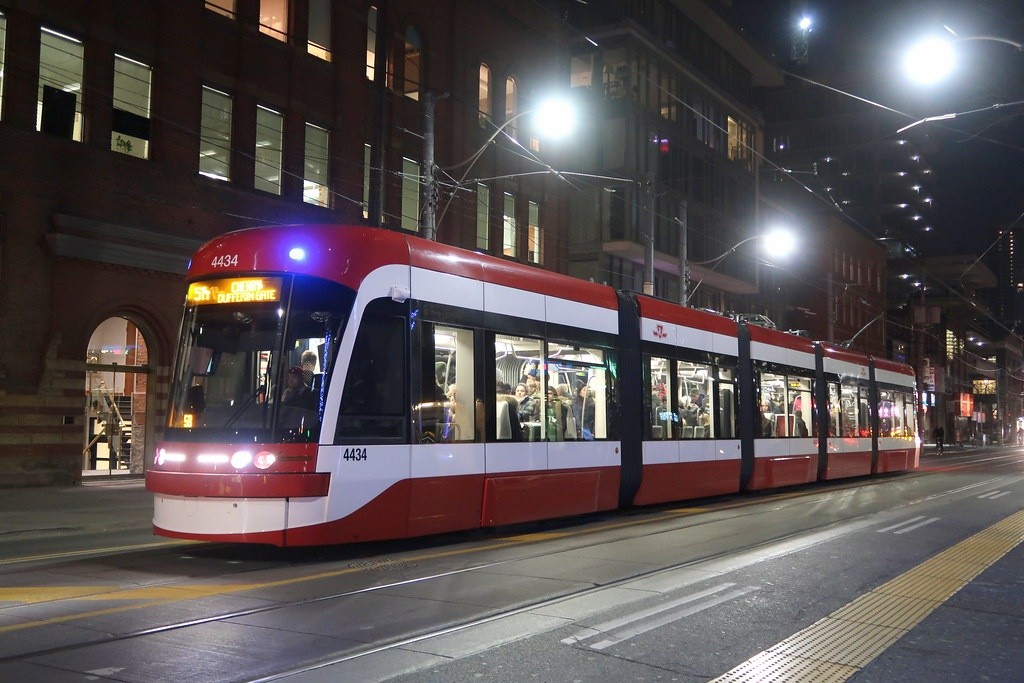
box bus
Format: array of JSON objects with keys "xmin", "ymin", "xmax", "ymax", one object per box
[{"xmin": 143, "ymin": 221, "xmax": 922, "ymax": 549}]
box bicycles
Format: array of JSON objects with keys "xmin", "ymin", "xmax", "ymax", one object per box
[
  {"xmin": 969, "ymin": 435, "xmax": 978, "ymax": 449},
  {"xmin": 944, "ymin": 436, "xmax": 962, "ymax": 448},
  {"xmin": 934, "ymin": 438, "xmax": 944, "ymax": 456},
  {"xmin": 1016, "ymin": 435, "xmax": 1024, "ymax": 447}
]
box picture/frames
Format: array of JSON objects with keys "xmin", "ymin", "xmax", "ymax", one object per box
[{"xmin": 86, "ymin": 349, "xmax": 102, "ymax": 378}]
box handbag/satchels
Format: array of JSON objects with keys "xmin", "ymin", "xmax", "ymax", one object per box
[{"xmin": 563, "ymin": 403, "xmax": 577, "ymax": 439}]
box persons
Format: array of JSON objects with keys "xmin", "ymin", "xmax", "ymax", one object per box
[
  {"xmin": 281, "ymin": 367, "xmax": 312, "ymax": 410},
  {"xmin": 760, "ymin": 390, "xmax": 808, "ymax": 437},
  {"xmin": 932, "ymin": 424, "xmax": 944, "ymax": 452},
  {"xmin": 548, "ymin": 383, "xmax": 595, "ymax": 437},
  {"xmin": 1016, "ymin": 428, "xmax": 1024, "ymax": 445},
  {"xmin": 651, "ymin": 380, "xmax": 709, "ymax": 438},
  {"xmin": 302, "ymin": 350, "xmax": 316, "ymax": 386},
  {"xmin": 435, "ymin": 362, "xmax": 530, "ymax": 442},
  {"xmin": 514, "ymin": 369, "xmax": 540, "ymax": 421}
]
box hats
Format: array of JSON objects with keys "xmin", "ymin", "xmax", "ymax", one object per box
[{"xmin": 689, "ymin": 388, "xmax": 700, "ymax": 396}]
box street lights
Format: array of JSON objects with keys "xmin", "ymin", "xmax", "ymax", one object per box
[
  {"xmin": 1008, "ymin": 410, "xmax": 1011, "ymax": 440},
  {"xmin": 682, "ymin": 227, "xmax": 799, "ymax": 307},
  {"xmin": 420, "ymin": 94, "xmax": 580, "ymax": 241}
]
